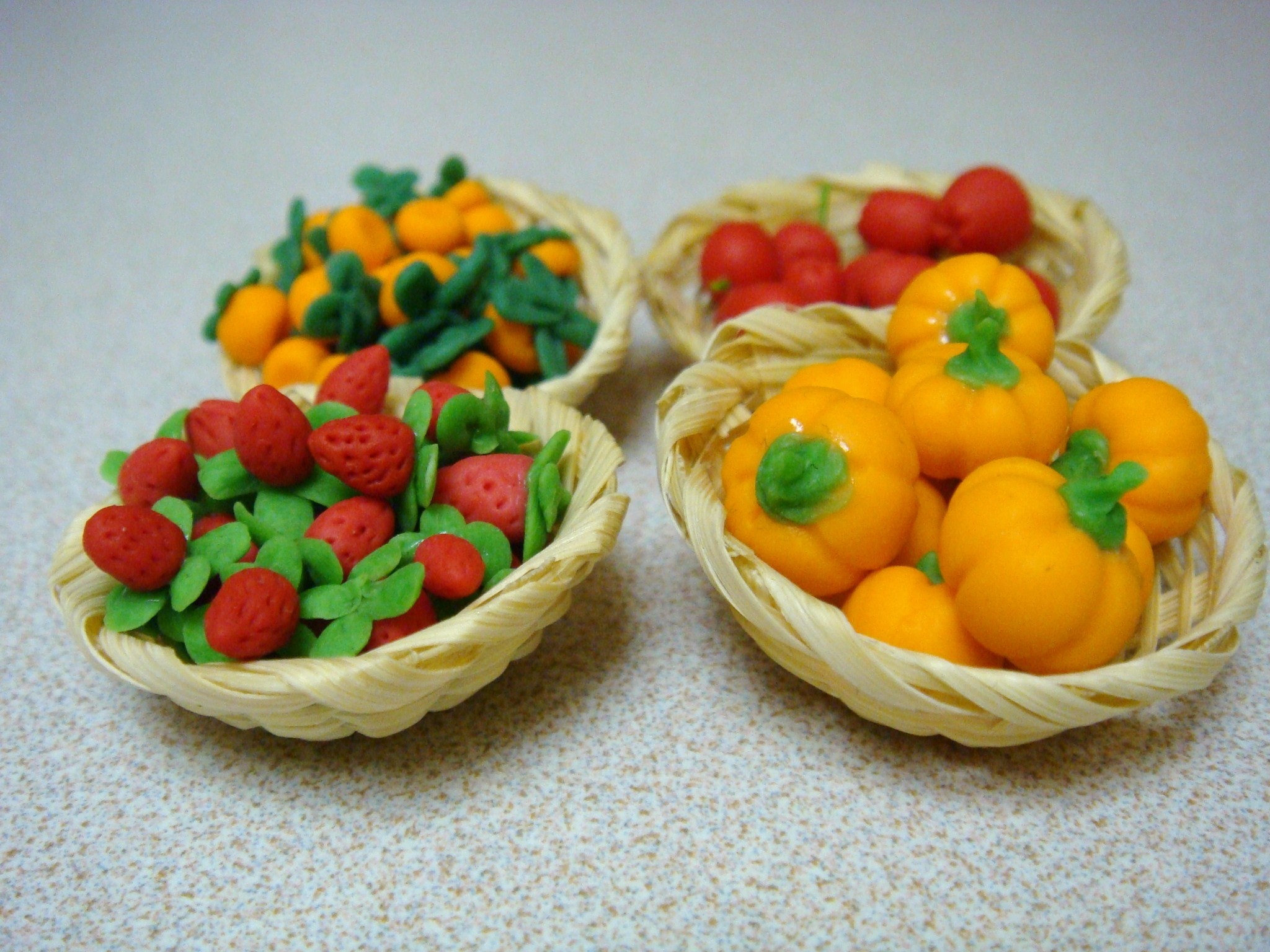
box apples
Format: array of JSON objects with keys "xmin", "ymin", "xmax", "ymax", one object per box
[{"xmin": 701, "ymin": 165, "xmax": 1060, "ymax": 336}]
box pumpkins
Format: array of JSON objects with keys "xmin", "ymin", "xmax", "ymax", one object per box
[{"xmin": 721, "ymin": 253, "xmax": 1209, "ymax": 673}]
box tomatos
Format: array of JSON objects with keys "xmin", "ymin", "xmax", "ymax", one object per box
[{"xmin": 200, "ymin": 154, "xmax": 598, "ymax": 392}]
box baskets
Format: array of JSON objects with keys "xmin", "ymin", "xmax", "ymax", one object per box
[
  {"xmin": 655, "ymin": 354, "xmax": 1269, "ymax": 749},
  {"xmin": 49, "ymin": 375, "xmax": 631, "ymax": 742},
  {"xmin": 640, "ymin": 165, "xmax": 1131, "ymax": 370},
  {"xmin": 229, "ymin": 180, "xmax": 642, "ymax": 406}
]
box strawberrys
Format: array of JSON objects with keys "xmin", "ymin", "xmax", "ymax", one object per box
[{"xmin": 83, "ymin": 345, "xmax": 571, "ymax": 662}]
